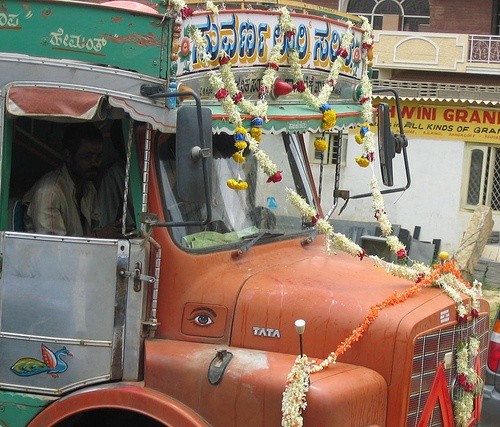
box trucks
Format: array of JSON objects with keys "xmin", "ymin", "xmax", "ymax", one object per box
[{"xmin": 0, "ymin": 0, "xmax": 490, "ymax": 427}]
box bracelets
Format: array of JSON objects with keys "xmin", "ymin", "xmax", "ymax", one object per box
[{"xmin": 94, "ymin": 230, "xmax": 98, "ymax": 238}]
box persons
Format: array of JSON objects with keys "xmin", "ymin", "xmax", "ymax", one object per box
[
  {"xmin": 25, "ymin": 121, "xmax": 135, "ymax": 238},
  {"xmin": 169, "ymin": 155, "xmax": 229, "ymax": 236},
  {"xmin": 96, "ymin": 125, "xmax": 146, "ymax": 239},
  {"xmin": 246, "ymin": 207, "xmax": 277, "ymax": 233}
]
